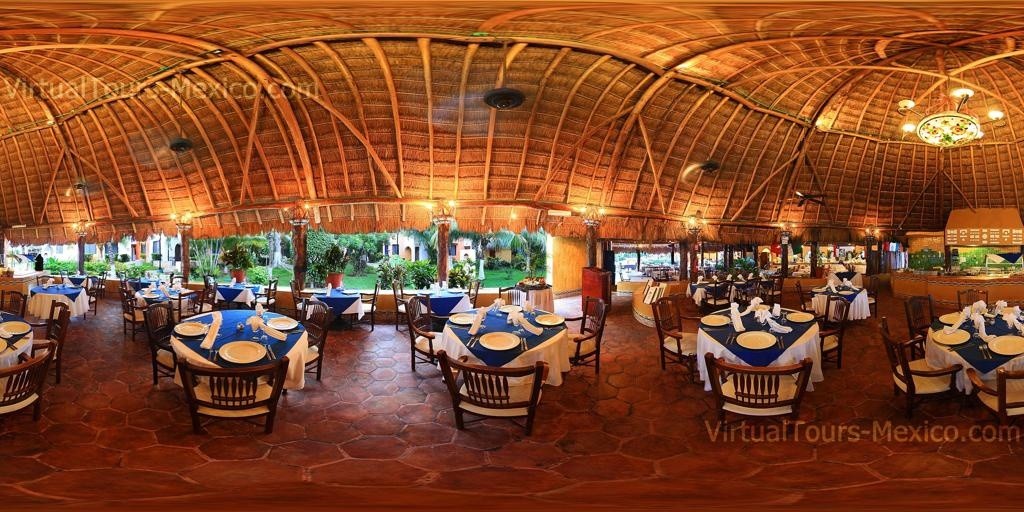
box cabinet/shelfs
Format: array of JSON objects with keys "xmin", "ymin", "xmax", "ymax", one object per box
[{"xmin": 582, "ymin": 265, "xmax": 611, "ymax": 315}]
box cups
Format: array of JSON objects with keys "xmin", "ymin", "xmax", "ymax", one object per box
[
  {"xmin": 261, "ymin": 327, "xmax": 269, "ymax": 344},
  {"xmin": 262, "ymin": 310, "xmax": 268, "ymax": 324}
]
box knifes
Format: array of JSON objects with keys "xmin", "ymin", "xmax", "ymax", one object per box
[
  {"xmin": 268, "ymin": 344, "xmax": 277, "ymax": 360},
  {"xmin": 520, "ymin": 337, "xmax": 525, "ymax": 351}
]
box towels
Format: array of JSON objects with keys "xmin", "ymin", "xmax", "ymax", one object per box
[
  {"xmin": 200, "ymin": 313, "xmax": 223, "ymax": 349},
  {"xmin": 748, "ymin": 273, "xmax": 753, "ymax": 280},
  {"xmin": 256, "ymin": 304, "xmax": 266, "ymax": 317},
  {"xmin": 767, "ymin": 319, "xmax": 793, "ymax": 334},
  {"xmin": 144, "ymin": 290, "xmax": 153, "ymax": 296},
  {"xmin": 830, "ymin": 283, "xmax": 836, "ymax": 293},
  {"xmin": 521, "ymin": 300, "xmax": 532, "ymax": 310},
  {"xmin": 64, "ymin": 278, "xmax": 74, "ymax": 286},
  {"xmin": 849, "ymin": 283, "xmax": 858, "ymax": 291},
  {"xmin": 433, "ymin": 283, "xmax": 439, "ymax": 291},
  {"xmin": 1014, "ymin": 306, "xmax": 1023, "ymax": 322},
  {"xmin": 161, "ymin": 286, "xmax": 171, "ymax": 297},
  {"xmin": 148, "ymin": 283, "xmax": 156, "ymax": 289},
  {"xmin": 252, "ymin": 318, "xmax": 286, "ymax": 341},
  {"xmin": 174, "ymin": 284, "xmax": 184, "ymax": 292},
  {"xmin": 442, "ymin": 281, "xmax": 447, "ymax": 289},
  {"xmin": 697, "ymin": 276, "xmax": 704, "ymax": 284},
  {"xmin": 943, "ymin": 317, "xmax": 965, "ymax": 334},
  {"xmin": 995, "ymin": 301, "xmax": 1005, "ymax": 313},
  {"xmin": 47, "ymin": 278, "xmax": 54, "ymax": 285},
  {"xmin": 727, "ymin": 275, "xmax": 733, "ymax": 281},
  {"xmin": 759, "ymin": 273, "xmax": 765, "ymax": 279},
  {"xmin": 326, "ymin": 283, "xmax": 332, "ymax": 296},
  {"xmin": 978, "ymin": 319, "xmax": 994, "ymax": 341},
  {"xmin": 841, "ymin": 279, "xmax": 848, "ymax": 286},
  {"xmin": 228, "ymin": 278, "xmax": 236, "ymax": 287},
  {"xmin": 731, "ymin": 303, "xmax": 745, "ymax": 331},
  {"xmin": 518, "ymin": 316, "xmax": 543, "ymax": 336},
  {"xmin": 712, "ymin": 275, "xmax": 720, "ymax": 282},
  {"xmin": 493, "ymin": 299, "xmax": 504, "ymax": 307},
  {"xmin": 468, "ymin": 307, "xmax": 485, "ymax": 335},
  {"xmin": 1010, "ymin": 315, "xmax": 1024, "ymax": 332},
  {"xmin": 737, "ymin": 275, "xmax": 746, "ymax": 281},
  {"xmin": 973, "ymin": 301, "xmax": 986, "ymax": 313},
  {"xmin": 436, "ymin": 287, "xmax": 442, "ymax": 297},
  {"xmin": 751, "ymin": 297, "xmax": 761, "ymax": 307},
  {"xmin": 773, "ymin": 304, "xmax": 781, "ymax": 315}
]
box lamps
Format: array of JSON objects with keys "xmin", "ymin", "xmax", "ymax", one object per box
[{"xmin": 896, "ymin": 87, "xmax": 1005, "ymax": 152}]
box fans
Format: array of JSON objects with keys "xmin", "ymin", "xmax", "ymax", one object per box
[
  {"xmin": 130, "ymin": 114, "xmax": 224, "ymax": 176},
  {"xmin": 681, "ymin": 157, "xmax": 726, "ymax": 191},
  {"xmin": 786, "ymin": 190, "xmax": 828, "ymax": 208},
  {"xmin": 47, "ymin": 176, "xmax": 110, "ymax": 199},
  {"xmin": 411, "ymin": 52, "xmax": 591, "ymax": 136}
]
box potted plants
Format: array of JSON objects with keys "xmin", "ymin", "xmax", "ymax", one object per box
[
  {"xmin": 323, "ymin": 242, "xmax": 347, "ymax": 289},
  {"xmin": 220, "ymin": 244, "xmax": 255, "ymax": 284}
]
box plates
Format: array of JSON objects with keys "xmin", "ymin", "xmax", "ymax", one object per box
[
  {"xmin": 266, "ymin": 317, "xmax": 299, "ymax": 331},
  {"xmin": 142, "ymin": 293, "xmax": 160, "ymax": 299},
  {"xmin": 735, "ymin": 330, "xmax": 777, "ymax": 350},
  {"xmin": 419, "ymin": 288, "xmax": 432, "ymax": 295},
  {"xmin": 498, "ymin": 305, "xmax": 523, "ymax": 313},
  {"xmin": 449, "ymin": 313, "xmax": 478, "ymax": 324},
  {"xmin": 218, "ymin": 340, "xmax": 267, "ymax": 364},
  {"xmin": 931, "ymin": 327, "xmax": 971, "ymax": 346},
  {"xmin": 785, "ymin": 312, "xmax": 815, "ymax": 323},
  {"xmin": 0, "ymin": 338, "xmax": 7, "ymax": 353},
  {"xmin": 173, "ymin": 321, "xmax": 209, "ymax": 336},
  {"xmin": 479, "ymin": 331, "xmax": 521, "ymax": 351},
  {"xmin": 535, "ymin": 313, "xmax": 564, "ymax": 326},
  {"xmin": 700, "ymin": 314, "xmax": 731, "ymax": 327},
  {"xmin": 169, "ymin": 294, "xmax": 184, "ymax": 299},
  {"xmin": 752, "ymin": 304, "xmax": 771, "ymax": 311},
  {"xmin": 987, "ymin": 335, "xmax": 1023, "ymax": 356},
  {"xmin": 1, "ymin": 321, "xmax": 32, "ymax": 335},
  {"xmin": 938, "ymin": 311, "xmax": 961, "ymax": 325},
  {"xmin": 811, "ymin": 288, "xmax": 827, "ymax": 293},
  {"xmin": 341, "ymin": 289, "xmax": 359, "ymax": 294},
  {"xmin": 448, "ymin": 288, "xmax": 463, "ymax": 293},
  {"xmin": 837, "ymin": 290, "xmax": 854, "ymax": 295}
]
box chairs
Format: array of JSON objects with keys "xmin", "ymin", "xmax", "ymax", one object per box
[
  {"xmin": 283, "ymin": 297, "xmax": 333, "ymax": 395},
  {"xmin": 391, "ymin": 278, "xmax": 416, "ymax": 331},
  {"xmin": 813, "ymin": 293, "xmax": 851, "ymax": 372},
  {"xmin": 178, "ymin": 289, "xmax": 206, "ymax": 323},
  {"xmin": 435, "ymin": 349, "xmax": 546, "ymax": 436},
  {"xmin": 253, "ymin": 278, "xmax": 280, "ymax": 312},
  {"xmin": 700, "ymin": 281, "xmax": 734, "ymax": 312},
  {"xmin": 965, "ymin": 366, "xmax": 1024, "ymax": 426},
  {"xmin": 0, "ymin": 339, "xmax": 58, "ymax": 422},
  {"xmin": 497, "ymin": 286, "xmax": 530, "ymax": 306},
  {"xmin": 957, "ymin": 290, "xmax": 990, "ymax": 314},
  {"xmin": 565, "ymin": 295, "xmax": 610, "ymax": 376},
  {"xmin": 877, "ymin": 316, "xmax": 964, "ymax": 423},
  {"xmin": 762, "ymin": 275, "xmax": 785, "ymax": 306},
  {"xmin": 92, "ymin": 271, "xmax": 108, "ymax": 299},
  {"xmin": 169, "ymin": 271, "xmax": 189, "ymax": 289},
  {"xmin": 903, "ymin": 294, "xmax": 936, "ymax": 362},
  {"xmin": 735, "ymin": 277, "xmax": 757, "ymax": 305},
  {"xmin": 36, "ymin": 275, "xmax": 55, "ymax": 288},
  {"xmin": 703, "ymin": 351, "xmax": 813, "ymax": 432},
  {"xmin": 289, "ymin": 279, "xmax": 308, "ymax": 320},
  {"xmin": 349, "ymin": 282, "xmax": 381, "ymax": 332},
  {"xmin": 466, "ymin": 281, "xmax": 480, "ymax": 308},
  {"xmin": 81, "ymin": 274, "xmax": 101, "ymax": 320},
  {"xmin": 652, "ymin": 294, "xmax": 705, "ymax": 385},
  {"xmin": 794, "ymin": 280, "xmax": 815, "ymax": 314},
  {"xmin": 0, "ymin": 289, "xmax": 28, "ymax": 319},
  {"xmin": 176, "ymin": 356, "xmax": 290, "ymax": 435},
  {"xmin": 195, "ymin": 282, "xmax": 217, "ymax": 313},
  {"xmin": 118, "ymin": 271, "xmax": 128, "ymax": 291},
  {"xmin": 142, "ymin": 299, "xmax": 178, "ymax": 385},
  {"xmin": 29, "ymin": 299, "xmax": 72, "ymax": 385},
  {"xmin": 116, "ymin": 285, "xmax": 144, "ymax": 341},
  {"xmin": 203, "ymin": 274, "xmax": 230, "ymax": 309},
  {"xmin": 866, "ymin": 276, "xmax": 880, "ymax": 317},
  {"xmin": 405, "ymin": 295, "xmax": 450, "ymax": 373},
  {"xmin": 60, "ymin": 269, "xmax": 68, "ymax": 282}
]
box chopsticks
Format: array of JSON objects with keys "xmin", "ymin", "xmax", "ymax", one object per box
[
  {"xmin": 8, "ymin": 345, "xmax": 15, "ymax": 350},
  {"xmin": 7, "ymin": 342, "xmax": 17, "ymax": 350}
]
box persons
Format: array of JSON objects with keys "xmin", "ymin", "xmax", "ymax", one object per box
[{"xmin": 464, "ymin": 253, "xmax": 477, "ymax": 277}]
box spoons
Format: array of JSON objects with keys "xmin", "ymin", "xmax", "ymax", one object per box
[
  {"xmin": 207, "ymin": 349, "xmax": 214, "ymax": 362},
  {"xmin": 466, "ymin": 335, "xmax": 475, "ymax": 347},
  {"xmin": 470, "ymin": 334, "xmax": 480, "ymax": 348},
  {"xmin": 982, "ymin": 343, "xmax": 992, "ymax": 360},
  {"xmin": 979, "ymin": 345, "xmax": 988, "ymax": 359}
]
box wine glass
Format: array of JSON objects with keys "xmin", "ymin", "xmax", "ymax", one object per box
[
  {"xmin": 781, "ymin": 311, "xmax": 787, "ymax": 326},
  {"xmin": 479, "ymin": 319, "xmax": 486, "ymax": 334},
  {"xmin": 517, "ymin": 320, "xmax": 525, "ymax": 336}
]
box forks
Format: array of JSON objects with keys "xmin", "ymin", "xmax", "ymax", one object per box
[
  {"xmin": 214, "ymin": 350, "xmax": 220, "ymax": 362},
  {"xmin": 261, "ymin": 336, "xmax": 272, "ymax": 360}
]
